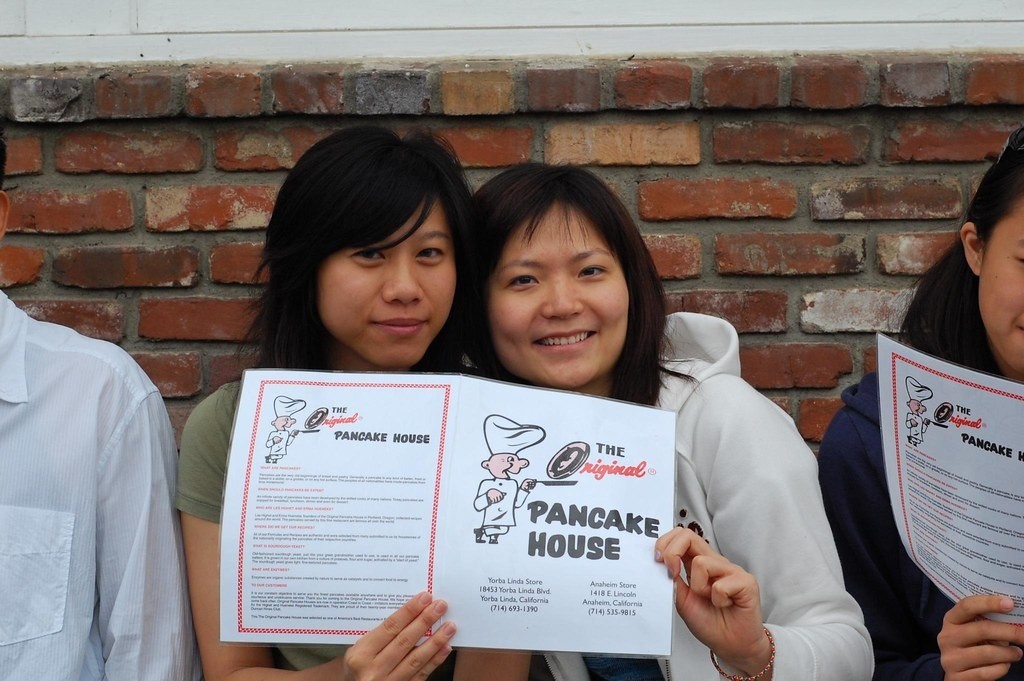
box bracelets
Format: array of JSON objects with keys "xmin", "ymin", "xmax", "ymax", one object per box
[{"xmin": 710, "ymin": 627, "xmax": 776, "ymax": 681}]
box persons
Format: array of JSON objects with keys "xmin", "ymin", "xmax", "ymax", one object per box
[
  {"xmin": 0, "ymin": 116, "xmax": 204, "ymax": 681},
  {"xmin": 175, "ymin": 123, "xmax": 874, "ymax": 681},
  {"xmin": 820, "ymin": 127, "xmax": 1024, "ymax": 681}
]
